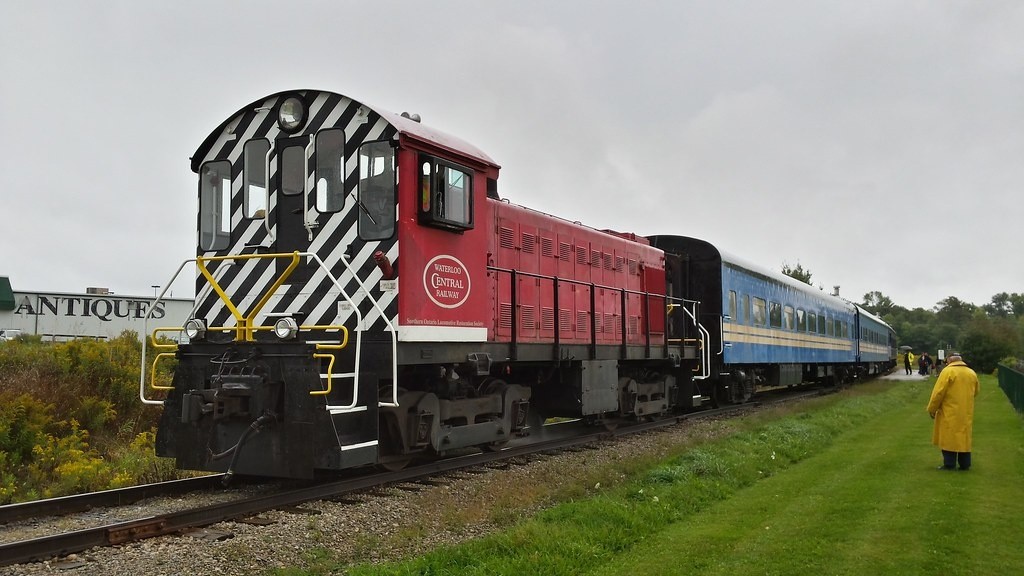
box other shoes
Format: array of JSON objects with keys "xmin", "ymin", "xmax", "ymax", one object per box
[
  {"xmin": 938, "ymin": 465, "xmax": 956, "ymax": 471},
  {"xmin": 957, "ymin": 465, "xmax": 970, "ymax": 470}
]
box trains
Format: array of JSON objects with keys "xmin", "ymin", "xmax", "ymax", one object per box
[{"xmin": 140, "ymin": 88, "xmax": 898, "ymax": 487}]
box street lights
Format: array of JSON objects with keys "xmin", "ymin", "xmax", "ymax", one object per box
[{"xmin": 152, "ymin": 285, "xmax": 160, "ymax": 297}]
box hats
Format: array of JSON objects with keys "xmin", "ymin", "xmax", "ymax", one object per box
[{"xmin": 946, "ymin": 351, "xmax": 963, "ymax": 358}]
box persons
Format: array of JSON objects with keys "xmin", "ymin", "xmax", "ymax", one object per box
[
  {"xmin": 926, "ymin": 351, "xmax": 980, "ymax": 472},
  {"xmin": 903, "ymin": 350, "xmax": 949, "ymax": 377}
]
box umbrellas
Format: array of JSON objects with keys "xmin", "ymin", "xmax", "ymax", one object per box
[{"xmin": 898, "ymin": 345, "xmax": 912, "ymax": 356}]
